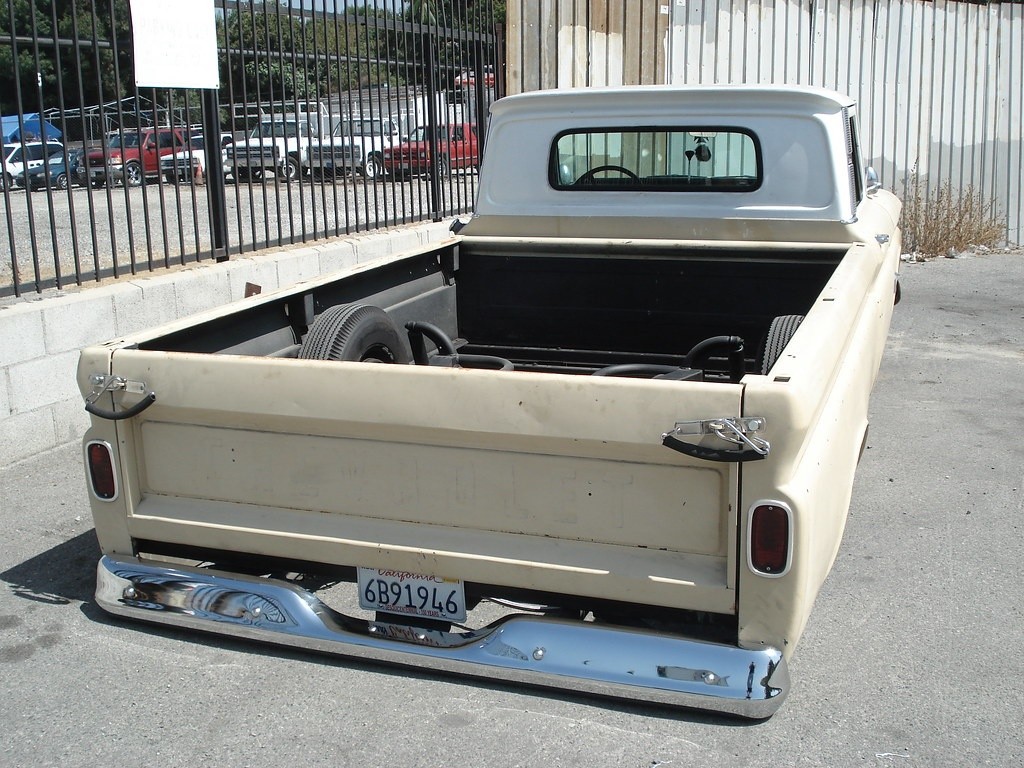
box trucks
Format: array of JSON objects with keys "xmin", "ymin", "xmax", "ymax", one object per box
[
  {"xmin": 225, "ymin": 119, "xmax": 325, "ymax": 184},
  {"xmin": 76, "ymin": 83, "xmax": 901, "ymax": 719},
  {"xmin": 304, "ymin": 117, "xmax": 422, "ymax": 180},
  {"xmin": 160, "ymin": 133, "xmax": 237, "ymax": 184}
]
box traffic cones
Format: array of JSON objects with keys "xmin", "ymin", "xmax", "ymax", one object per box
[{"xmin": 194, "ymin": 162, "xmax": 206, "ymax": 187}]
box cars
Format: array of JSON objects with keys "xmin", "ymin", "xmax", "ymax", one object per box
[
  {"xmin": 455, "ymin": 65, "xmax": 494, "ymax": 89},
  {"xmin": 16, "ymin": 146, "xmax": 86, "ymax": 192},
  {"xmin": 0, "ymin": 138, "xmax": 72, "ymax": 191},
  {"xmin": 384, "ymin": 123, "xmax": 479, "ymax": 182},
  {"xmin": 77, "ymin": 129, "xmax": 201, "ymax": 189}
]
